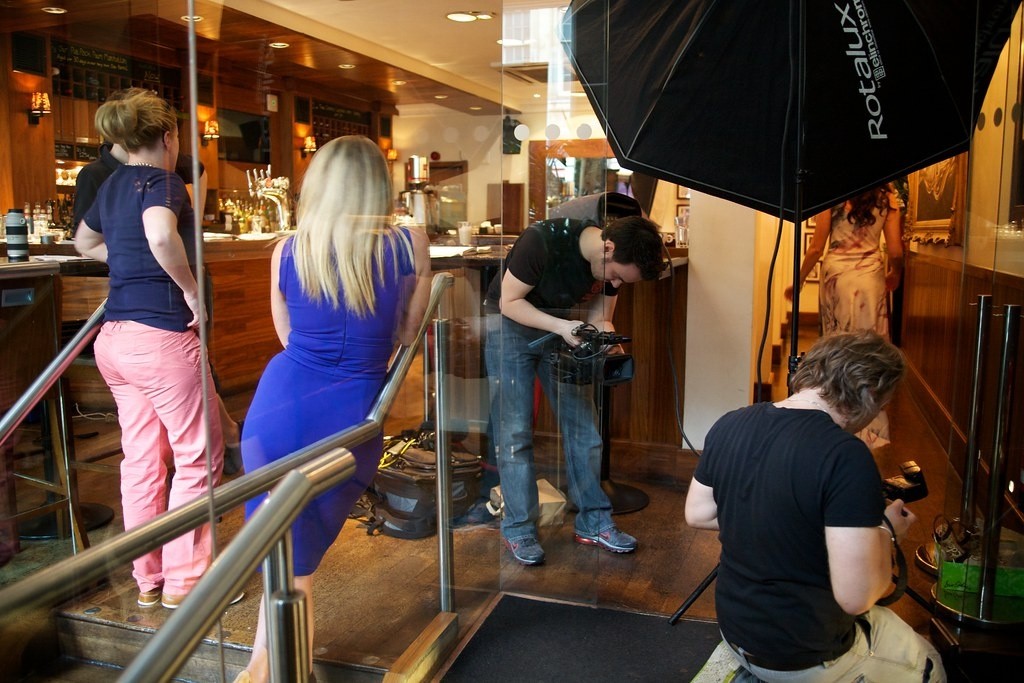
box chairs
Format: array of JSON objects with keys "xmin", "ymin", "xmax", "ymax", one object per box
[{"xmin": 0, "ymin": 260, "xmax": 91, "ymax": 569}]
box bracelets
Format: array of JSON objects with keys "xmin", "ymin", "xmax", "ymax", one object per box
[{"xmin": 876, "ymin": 526, "xmax": 895, "ymax": 542}]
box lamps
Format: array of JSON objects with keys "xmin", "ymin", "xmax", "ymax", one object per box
[
  {"xmin": 28, "ymin": 92, "xmax": 52, "ymax": 124},
  {"xmin": 202, "ymin": 120, "xmax": 220, "ymax": 146},
  {"xmin": 387, "ymin": 148, "xmax": 397, "ymax": 161},
  {"xmin": 301, "ymin": 136, "xmax": 317, "ymax": 157}
]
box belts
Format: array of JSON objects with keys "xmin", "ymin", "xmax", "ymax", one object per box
[{"xmin": 722, "ymin": 636, "xmax": 824, "ymax": 672}]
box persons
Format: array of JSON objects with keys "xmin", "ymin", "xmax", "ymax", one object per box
[
  {"xmin": 485, "ymin": 216, "xmax": 664, "ymax": 566},
  {"xmin": 234, "ymin": 134, "xmax": 432, "ymax": 683},
  {"xmin": 783, "ymin": 181, "xmax": 903, "ymax": 449},
  {"xmin": 685, "ymin": 324, "xmax": 948, "ymax": 683},
  {"xmin": 74, "ymin": 86, "xmax": 157, "ymax": 236},
  {"xmin": 74, "ymin": 91, "xmax": 245, "ymax": 609}
]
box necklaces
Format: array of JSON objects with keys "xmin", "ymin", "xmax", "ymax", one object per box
[{"xmin": 125, "ymin": 162, "xmax": 158, "ymax": 167}]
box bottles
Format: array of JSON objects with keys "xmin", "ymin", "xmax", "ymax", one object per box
[
  {"xmin": 5, "ymin": 208, "xmax": 30, "ymax": 263},
  {"xmin": 219, "ymin": 199, "xmax": 279, "ymax": 234},
  {"xmin": 25, "ymin": 193, "xmax": 74, "ymax": 242},
  {"xmin": 674, "ymin": 216, "xmax": 689, "ymax": 248},
  {"xmin": 412, "ymin": 190, "xmax": 439, "ymax": 224}
]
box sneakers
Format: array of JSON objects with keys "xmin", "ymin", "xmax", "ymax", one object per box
[
  {"xmin": 576, "ymin": 527, "xmax": 637, "ymax": 553},
  {"xmin": 502, "ymin": 536, "xmax": 545, "ymax": 565}
]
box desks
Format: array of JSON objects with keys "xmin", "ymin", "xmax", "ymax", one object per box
[{"xmin": 424, "ymin": 245, "xmax": 691, "ymax": 477}]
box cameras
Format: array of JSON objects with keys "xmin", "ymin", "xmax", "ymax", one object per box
[{"xmin": 879, "ymin": 460, "xmax": 929, "ymax": 518}]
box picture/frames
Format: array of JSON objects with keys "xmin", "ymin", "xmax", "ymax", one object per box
[
  {"xmin": 804, "ymin": 233, "xmax": 824, "ymax": 256},
  {"xmin": 677, "ymin": 183, "xmax": 691, "ymax": 200},
  {"xmin": 676, "ymin": 204, "xmax": 690, "ymax": 218},
  {"xmin": 805, "ymin": 261, "xmax": 822, "ymax": 284},
  {"xmin": 806, "ymin": 215, "xmax": 817, "ymax": 228},
  {"xmin": 900, "ymin": 149, "xmax": 970, "ymax": 247}
]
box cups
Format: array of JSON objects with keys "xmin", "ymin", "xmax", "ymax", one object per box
[
  {"xmin": 458, "ymin": 221, "xmax": 471, "ymax": 245},
  {"xmin": 41, "ymin": 236, "xmax": 54, "ymax": 244},
  {"xmin": 402, "ymin": 192, "xmax": 410, "ymax": 208}
]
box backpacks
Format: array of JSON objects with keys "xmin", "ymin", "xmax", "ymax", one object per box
[{"xmin": 353, "ymin": 430, "xmax": 485, "ymax": 535}]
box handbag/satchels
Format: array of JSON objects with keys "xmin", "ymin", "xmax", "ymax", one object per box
[{"xmin": 487, "ymin": 478, "xmax": 566, "ymax": 526}]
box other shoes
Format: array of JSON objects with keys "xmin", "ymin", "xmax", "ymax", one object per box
[
  {"xmin": 161, "ymin": 589, "xmax": 246, "ymax": 608},
  {"xmin": 138, "ymin": 587, "xmax": 162, "ymax": 605}
]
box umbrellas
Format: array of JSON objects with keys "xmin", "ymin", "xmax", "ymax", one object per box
[{"xmin": 560, "ymin": 0, "xmax": 1024, "ymax": 395}]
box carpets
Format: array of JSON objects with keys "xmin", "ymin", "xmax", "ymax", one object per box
[{"xmin": 430, "ymin": 590, "xmax": 723, "ymax": 683}]
